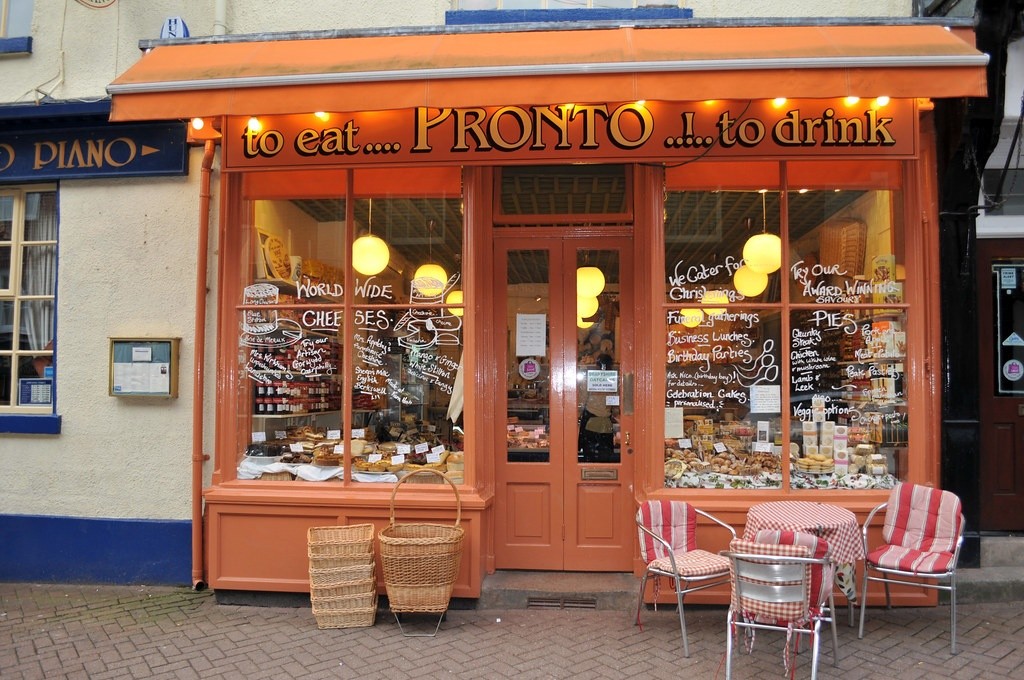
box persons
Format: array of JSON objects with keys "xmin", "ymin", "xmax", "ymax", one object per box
[{"xmin": 576, "ymin": 354, "xmax": 620, "ymax": 463}]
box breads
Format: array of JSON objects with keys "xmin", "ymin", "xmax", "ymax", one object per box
[
  {"xmin": 848, "ymin": 444, "xmax": 888, "ymax": 476},
  {"xmin": 294, "ymin": 431, "xmax": 449, "ymax": 472},
  {"xmin": 665, "ymin": 438, "xmax": 834, "ymax": 476}
]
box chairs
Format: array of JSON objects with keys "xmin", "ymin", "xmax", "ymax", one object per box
[
  {"xmin": 719, "ymin": 539, "xmax": 838, "ymax": 680},
  {"xmin": 858, "ymin": 482, "xmax": 966, "ymax": 655},
  {"xmin": 633, "ymin": 500, "xmax": 752, "ymax": 658}
]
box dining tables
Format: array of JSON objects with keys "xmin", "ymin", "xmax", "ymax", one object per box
[{"xmin": 743, "ymin": 501, "xmax": 866, "ymax": 652}]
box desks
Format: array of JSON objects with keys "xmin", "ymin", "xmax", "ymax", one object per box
[
  {"xmin": 507, "ymin": 448, "xmax": 549, "ymax": 463},
  {"xmin": 506, "ymin": 399, "xmax": 550, "ymax": 425}
]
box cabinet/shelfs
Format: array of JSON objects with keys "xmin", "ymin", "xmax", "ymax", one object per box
[
  {"xmin": 382, "ymin": 354, "xmax": 424, "ymax": 422},
  {"xmin": 836, "ymin": 312, "xmax": 907, "ymax": 482},
  {"xmin": 252, "ymin": 278, "xmax": 375, "ymax": 419}
]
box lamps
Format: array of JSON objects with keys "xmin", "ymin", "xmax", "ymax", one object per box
[
  {"xmin": 680, "ymin": 278, "xmax": 703, "ymax": 327},
  {"xmin": 577, "ymin": 250, "xmax": 605, "ymax": 329},
  {"xmin": 701, "ymin": 253, "xmax": 729, "ymax": 316},
  {"xmin": 743, "ymin": 192, "xmax": 782, "ymax": 273},
  {"xmin": 352, "ymin": 198, "xmax": 390, "ymax": 275},
  {"xmin": 445, "ymin": 254, "xmax": 464, "ymax": 317},
  {"xmin": 414, "ymin": 217, "xmax": 447, "ymax": 297},
  {"xmin": 733, "ymin": 215, "xmax": 768, "ymax": 297}
]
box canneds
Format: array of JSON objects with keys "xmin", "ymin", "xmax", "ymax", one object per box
[
  {"xmin": 255, "ymin": 339, "xmax": 275, "ymax": 370},
  {"xmin": 255, "ymin": 381, "xmax": 330, "ymax": 415}
]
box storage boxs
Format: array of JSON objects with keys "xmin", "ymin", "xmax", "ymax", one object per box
[
  {"xmin": 818, "ymin": 218, "xmax": 868, "ymax": 278},
  {"xmin": 307, "ymin": 523, "xmax": 379, "ymax": 629},
  {"xmin": 378, "ymin": 468, "xmax": 465, "ymax": 637},
  {"xmin": 108, "ymin": 336, "xmax": 182, "ymax": 398}
]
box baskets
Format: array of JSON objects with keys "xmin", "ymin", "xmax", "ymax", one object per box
[
  {"xmin": 796, "ymin": 252, "xmax": 826, "ymax": 295},
  {"xmin": 819, "ymin": 217, "xmax": 867, "ymax": 279},
  {"xmin": 377, "ymin": 469, "xmax": 467, "ymax": 612},
  {"xmin": 307, "ymin": 523, "xmax": 377, "ymax": 630},
  {"xmin": 254, "ymin": 465, "xmax": 466, "ymax": 483}
]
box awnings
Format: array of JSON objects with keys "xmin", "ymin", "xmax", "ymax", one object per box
[{"xmin": 100, "ymin": 23, "xmax": 992, "ymax": 123}]
